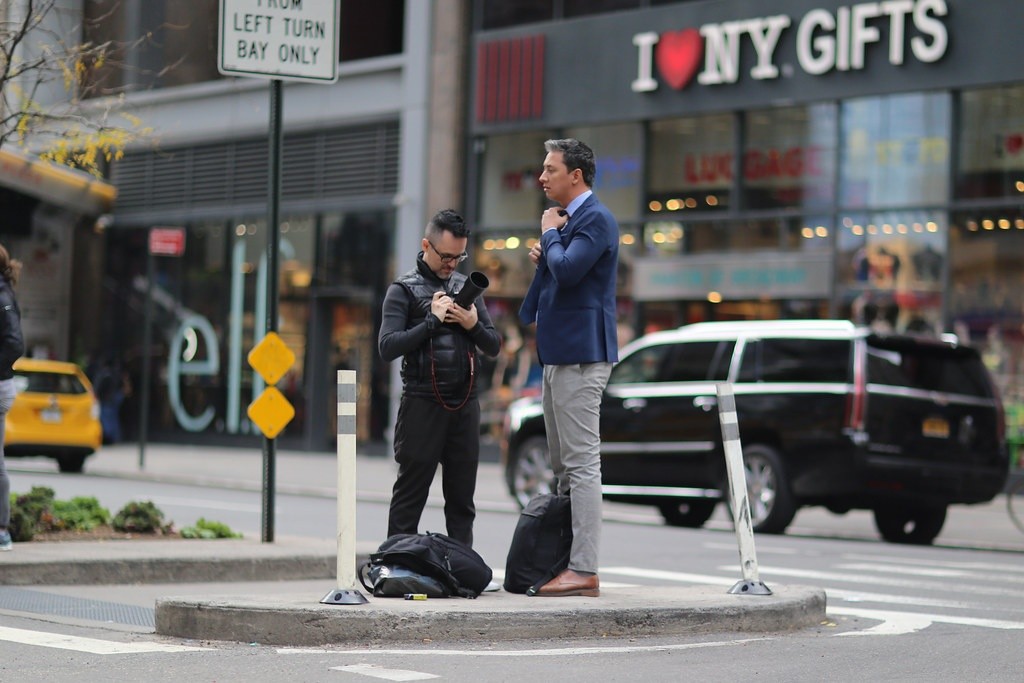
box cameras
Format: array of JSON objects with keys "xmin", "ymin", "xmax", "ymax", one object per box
[{"xmin": 442, "ymin": 271, "xmax": 490, "ymax": 327}]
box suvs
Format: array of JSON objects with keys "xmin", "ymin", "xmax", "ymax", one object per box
[{"xmin": 503, "ymin": 319, "xmax": 1003, "ymax": 544}]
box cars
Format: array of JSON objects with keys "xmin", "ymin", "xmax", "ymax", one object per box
[{"xmin": 6, "ymin": 357, "xmax": 103, "ymax": 473}]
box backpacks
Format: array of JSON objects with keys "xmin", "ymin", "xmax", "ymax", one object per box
[
  {"xmin": 358, "ymin": 529, "xmax": 493, "ymax": 600},
  {"xmin": 503, "ymin": 475, "xmax": 576, "ymax": 594}
]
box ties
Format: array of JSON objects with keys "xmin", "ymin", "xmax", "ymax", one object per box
[{"xmin": 517, "ymin": 210, "xmax": 568, "ymax": 326}]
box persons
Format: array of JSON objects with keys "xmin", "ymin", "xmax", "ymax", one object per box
[
  {"xmin": 516, "ymin": 137, "xmax": 623, "ymax": 596},
  {"xmin": 377, "ymin": 207, "xmax": 503, "ymax": 553}
]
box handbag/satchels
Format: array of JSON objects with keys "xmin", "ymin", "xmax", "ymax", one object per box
[{"xmin": 367, "ymin": 559, "xmax": 449, "ymax": 597}]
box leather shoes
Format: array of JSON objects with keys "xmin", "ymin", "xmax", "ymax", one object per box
[{"xmin": 538, "ymin": 567, "xmax": 601, "ymax": 596}]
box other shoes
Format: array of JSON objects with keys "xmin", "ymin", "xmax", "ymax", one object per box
[{"xmin": 0, "ymin": 531, "xmax": 16, "ymax": 553}]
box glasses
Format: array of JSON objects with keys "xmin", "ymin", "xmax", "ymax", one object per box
[{"xmin": 428, "ymin": 239, "xmax": 468, "ymax": 264}]
